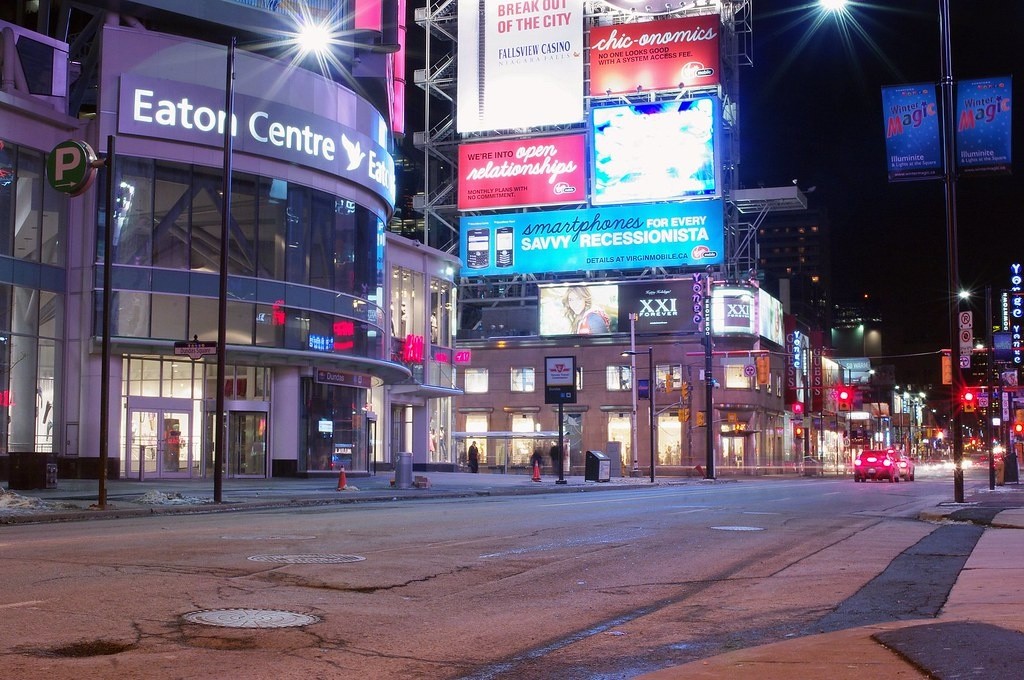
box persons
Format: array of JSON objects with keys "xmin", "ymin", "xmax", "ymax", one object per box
[
  {"xmin": 561, "ymin": 287, "xmax": 610, "ymax": 333},
  {"xmin": 469, "ymin": 441, "xmax": 479, "ymax": 473},
  {"xmin": 430, "ymin": 424, "xmax": 448, "ymax": 463}
]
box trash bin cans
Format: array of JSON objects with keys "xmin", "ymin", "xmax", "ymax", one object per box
[
  {"xmin": 394, "ymin": 451, "xmax": 413, "ymax": 489},
  {"xmin": 585, "ymin": 450, "xmax": 611, "ymax": 483},
  {"xmin": 809, "ymin": 458, "xmax": 820, "ymax": 476},
  {"xmin": 1002, "ymin": 451, "xmax": 1019, "ymax": 484}
]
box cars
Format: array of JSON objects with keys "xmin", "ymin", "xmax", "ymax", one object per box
[
  {"xmin": 804, "ymin": 456, "xmax": 821, "ymax": 475},
  {"xmin": 854, "ymin": 448, "xmax": 901, "ymax": 482},
  {"xmin": 882, "ymin": 449, "xmax": 915, "ymax": 482},
  {"xmin": 913, "ymin": 440, "xmax": 1013, "ymax": 476}
]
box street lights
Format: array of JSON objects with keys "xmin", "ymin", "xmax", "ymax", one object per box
[
  {"xmin": 214, "ymin": 1, "xmax": 339, "ymax": 505},
  {"xmin": 619, "ymin": 347, "xmax": 654, "ymax": 484}
]
